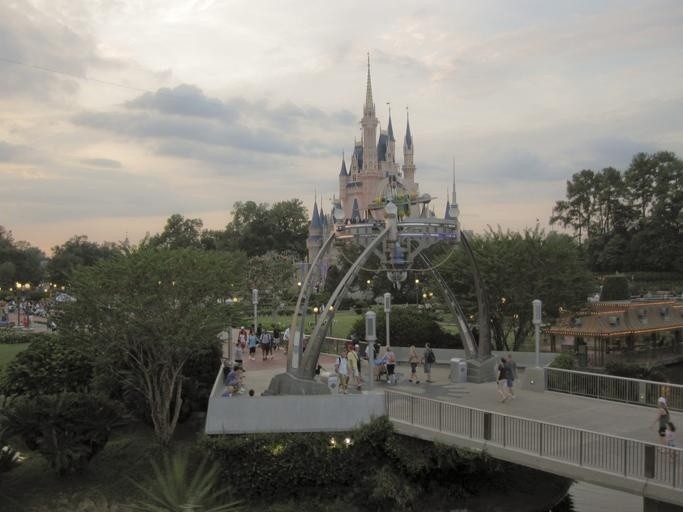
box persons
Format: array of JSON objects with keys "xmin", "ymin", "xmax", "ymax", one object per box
[
  {"xmin": 664, "ymin": 421, "xmax": 676, "ymax": 447},
  {"xmin": 2, "ymin": 300, "xmax": 55, "ymax": 328},
  {"xmin": 224, "ymin": 324, "xmax": 292, "ymax": 398},
  {"xmin": 650, "ymin": 397, "xmax": 669, "ymax": 443},
  {"xmin": 496, "ymin": 357, "xmax": 510, "ymax": 401},
  {"xmin": 504, "ymin": 355, "xmax": 518, "ymax": 398},
  {"xmin": 315, "ymin": 342, "xmax": 434, "ymax": 393}
]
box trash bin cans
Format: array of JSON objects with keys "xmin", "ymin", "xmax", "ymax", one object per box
[
  {"xmin": 449, "ymin": 357, "xmax": 468, "ymax": 383},
  {"xmin": 320, "ymin": 371, "xmax": 339, "ymax": 394}
]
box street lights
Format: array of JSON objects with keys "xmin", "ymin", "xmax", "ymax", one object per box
[
  {"xmin": 532, "ymin": 299, "xmax": 542, "ymax": 368},
  {"xmin": 384, "ymin": 293, "xmax": 391, "ymax": 347},
  {"xmin": 314, "ymin": 306, "xmax": 333, "ymax": 337},
  {"xmin": 15, "ymin": 281, "xmax": 30, "ymax": 325},
  {"xmin": 365, "ymin": 311, "xmax": 377, "ymax": 390},
  {"xmin": 415, "ymin": 279, "xmax": 419, "ymax": 307},
  {"xmin": 252, "ymin": 289, "xmax": 259, "ymax": 334}
]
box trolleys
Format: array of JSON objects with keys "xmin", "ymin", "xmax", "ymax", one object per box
[{"xmin": 374, "ymin": 362, "xmax": 389, "ymax": 381}]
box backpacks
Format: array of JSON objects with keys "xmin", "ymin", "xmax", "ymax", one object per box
[
  {"xmin": 388, "ymin": 355, "xmax": 396, "ymax": 364},
  {"xmin": 428, "ymin": 349, "xmax": 435, "ymax": 363}
]
